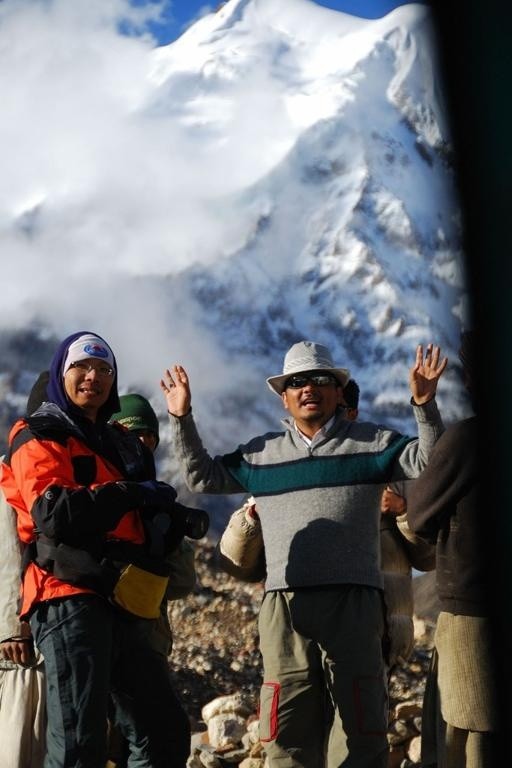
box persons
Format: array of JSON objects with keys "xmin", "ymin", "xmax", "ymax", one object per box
[
  {"xmin": 0, "ymin": 331, "xmax": 208, "ymax": 768},
  {"xmin": 161, "ymin": 331, "xmax": 512, "ymax": 768}
]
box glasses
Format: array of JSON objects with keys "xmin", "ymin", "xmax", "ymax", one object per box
[
  {"xmin": 285, "ymin": 373, "xmax": 334, "ymax": 388},
  {"xmin": 73, "ymin": 361, "xmax": 113, "ymax": 376}
]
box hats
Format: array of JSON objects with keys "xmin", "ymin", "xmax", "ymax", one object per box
[
  {"xmin": 107, "ymin": 394, "xmax": 159, "ymax": 451},
  {"xmin": 266, "ymin": 341, "xmax": 350, "ymax": 396},
  {"xmin": 62, "ymin": 334, "xmax": 113, "ymax": 377}
]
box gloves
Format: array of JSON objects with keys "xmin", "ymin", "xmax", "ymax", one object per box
[{"xmin": 116, "ymin": 480, "xmax": 176, "ymax": 508}]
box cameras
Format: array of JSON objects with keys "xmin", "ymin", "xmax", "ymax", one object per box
[{"xmin": 162, "ymin": 500, "xmax": 211, "ymax": 555}]
